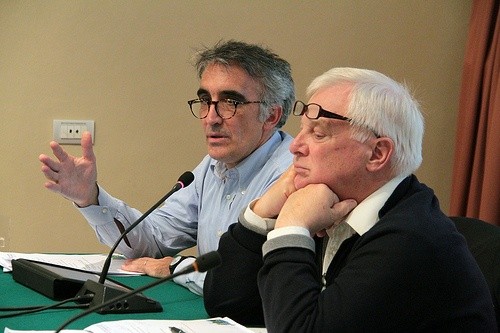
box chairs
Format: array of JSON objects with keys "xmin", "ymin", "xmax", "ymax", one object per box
[{"xmin": 449, "ymin": 216, "xmax": 500, "ymax": 330}]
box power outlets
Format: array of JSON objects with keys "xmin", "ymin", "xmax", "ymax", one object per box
[{"xmin": 52, "ymin": 120, "xmax": 95, "ymax": 144}]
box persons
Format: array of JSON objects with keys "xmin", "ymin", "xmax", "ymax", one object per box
[
  {"xmin": 203, "ymin": 67, "xmax": 496, "ymax": 333},
  {"xmin": 38, "ymin": 40, "xmax": 297, "ymax": 297}
]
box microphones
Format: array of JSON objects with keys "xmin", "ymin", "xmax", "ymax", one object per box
[
  {"xmin": 55, "ymin": 251, "xmax": 223, "ymax": 333},
  {"xmin": 73, "ymin": 169, "xmax": 195, "ymax": 314}
]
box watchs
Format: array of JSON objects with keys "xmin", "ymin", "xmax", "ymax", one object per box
[{"xmin": 167, "ymin": 254, "xmax": 197, "ymax": 274}]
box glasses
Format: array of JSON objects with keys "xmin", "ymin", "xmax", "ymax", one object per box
[
  {"xmin": 293, "ymin": 100, "xmax": 381, "ymax": 138},
  {"xmin": 187, "ymin": 97, "xmax": 269, "ymax": 120}
]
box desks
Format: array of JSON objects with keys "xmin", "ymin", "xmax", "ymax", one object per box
[{"xmin": 0, "ymin": 253, "xmax": 265, "ymax": 333}]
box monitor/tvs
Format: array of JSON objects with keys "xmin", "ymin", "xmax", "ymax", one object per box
[{"xmin": 11, "ymin": 258, "xmax": 147, "ymax": 302}]
